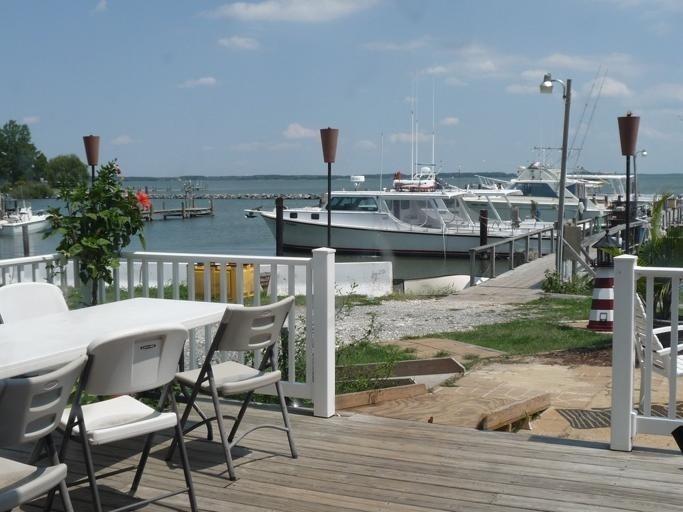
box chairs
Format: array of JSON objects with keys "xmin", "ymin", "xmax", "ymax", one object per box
[
  {"xmin": 165, "ymin": 296, "xmax": 298, "ymax": 481},
  {"xmin": 634, "ymin": 291, "xmax": 683, "ymax": 420},
  {"xmin": 44, "ymin": 325, "xmax": 198, "ymax": 512},
  {"xmin": 1, "ymin": 355, "xmax": 76, "ymax": 512},
  {"xmin": 0, "ymin": 282, "xmax": 69, "ymax": 323}
]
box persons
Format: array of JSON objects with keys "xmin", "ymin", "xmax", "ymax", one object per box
[
  {"xmin": 530, "ymin": 198, "xmax": 535, "ymax": 218},
  {"xmin": 511, "ymin": 206, "xmax": 520, "ymax": 228},
  {"xmin": 536, "ymin": 207, "xmax": 541, "ymax": 223}
]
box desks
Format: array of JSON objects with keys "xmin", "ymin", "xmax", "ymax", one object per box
[{"xmin": 0, "ymin": 296, "xmax": 245, "ymax": 436}]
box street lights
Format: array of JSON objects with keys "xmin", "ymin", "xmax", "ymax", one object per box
[
  {"xmin": 320, "ymin": 127, "xmax": 339, "ymax": 247},
  {"xmin": 541, "ymin": 73, "xmax": 571, "ymax": 290},
  {"xmin": 618, "ymin": 112, "xmax": 640, "ymax": 254},
  {"xmin": 83, "ymin": 135, "xmax": 99, "ymax": 185}
]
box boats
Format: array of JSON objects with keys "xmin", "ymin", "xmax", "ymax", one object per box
[{"xmin": 0, "ymin": 207, "xmax": 54, "ymax": 235}]
